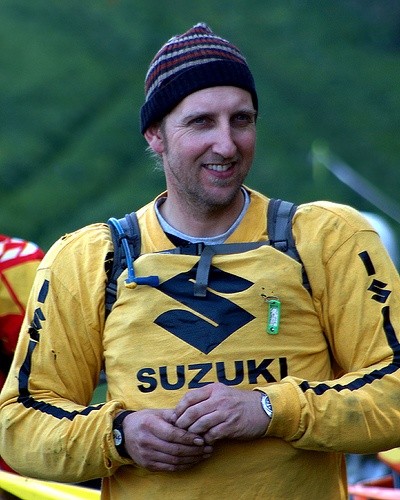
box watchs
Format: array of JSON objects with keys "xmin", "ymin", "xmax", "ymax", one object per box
[{"xmin": 259, "ymin": 393, "xmax": 272, "ymax": 419}]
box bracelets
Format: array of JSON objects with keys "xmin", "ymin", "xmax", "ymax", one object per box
[{"xmin": 113, "ymin": 410, "xmax": 136, "ymax": 459}]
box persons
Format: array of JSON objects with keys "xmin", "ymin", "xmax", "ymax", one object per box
[{"xmin": 0, "ymin": 23, "xmax": 400, "ymax": 500}]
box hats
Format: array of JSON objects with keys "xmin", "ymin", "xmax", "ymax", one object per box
[{"xmin": 140, "ymin": 22, "xmax": 259, "ymax": 135}]
